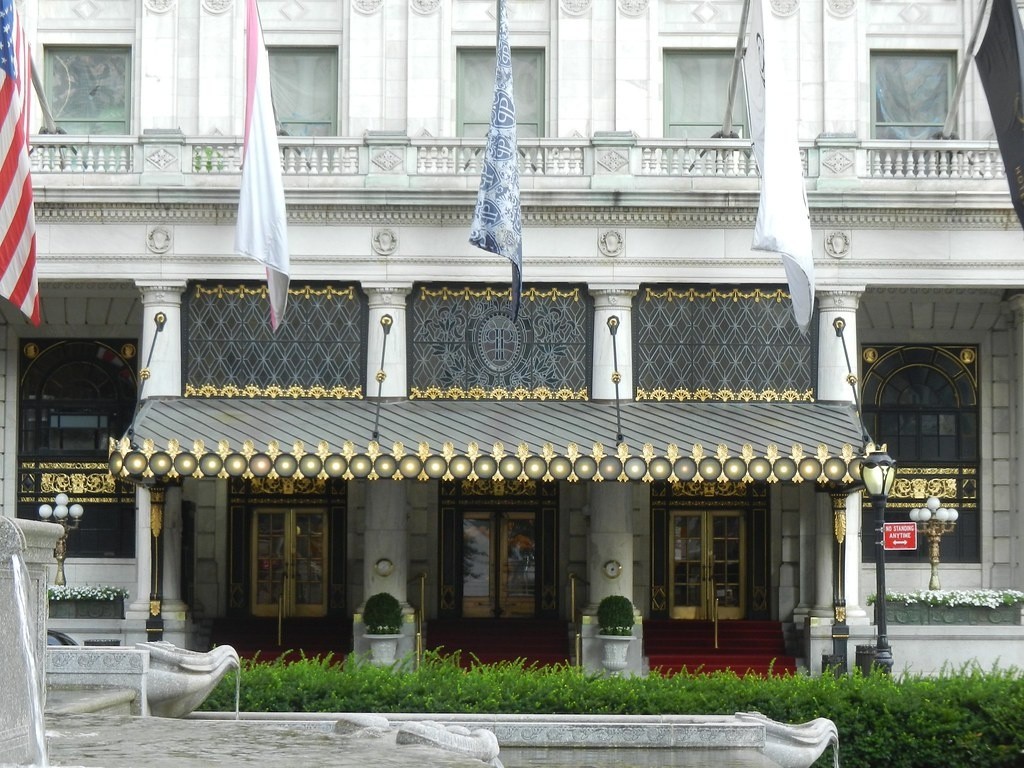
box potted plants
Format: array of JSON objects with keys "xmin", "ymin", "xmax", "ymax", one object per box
[
  {"xmin": 594, "ymin": 596, "xmax": 637, "ymax": 670},
  {"xmin": 361, "ymin": 592, "xmax": 404, "ymax": 667}
]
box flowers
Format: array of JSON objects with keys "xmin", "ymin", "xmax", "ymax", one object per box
[
  {"xmin": 46, "ymin": 583, "xmax": 131, "ymax": 599},
  {"xmin": 867, "ymin": 588, "xmax": 1024, "ymax": 610}
]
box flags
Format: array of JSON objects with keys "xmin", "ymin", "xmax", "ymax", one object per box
[
  {"xmin": 468, "ymin": 0, "xmax": 523, "ymax": 322},
  {"xmin": 741, "ymin": 0, "xmax": 817, "ymax": 337},
  {"xmin": 0, "ymin": 0, "xmax": 43, "ymax": 328},
  {"xmin": 233, "ymin": 1, "xmax": 290, "ymax": 333},
  {"xmin": 971, "ymin": 0, "xmax": 1024, "ymax": 235}
]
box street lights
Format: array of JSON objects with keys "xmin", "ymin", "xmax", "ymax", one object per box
[
  {"xmin": 865, "ymin": 450, "xmax": 898, "ymax": 679},
  {"xmin": 910, "ymin": 496, "xmax": 958, "ymax": 591},
  {"xmin": 38, "ymin": 491, "xmax": 86, "ymax": 587}
]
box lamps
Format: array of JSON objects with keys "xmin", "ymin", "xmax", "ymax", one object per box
[
  {"xmin": 910, "ymin": 497, "xmax": 959, "ymax": 590},
  {"xmin": 39, "ymin": 493, "xmax": 84, "ymax": 585}
]
